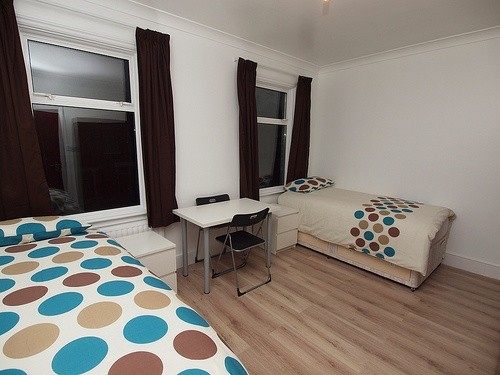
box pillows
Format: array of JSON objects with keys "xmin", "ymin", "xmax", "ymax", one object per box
[
  {"xmin": 283, "ymin": 176, "xmax": 335, "ymax": 193},
  {"xmin": 0, "ymin": 215, "xmax": 93, "ymax": 247}
]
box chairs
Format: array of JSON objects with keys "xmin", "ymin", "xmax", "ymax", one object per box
[{"xmin": 195, "ymin": 193, "xmax": 271, "ymax": 297}]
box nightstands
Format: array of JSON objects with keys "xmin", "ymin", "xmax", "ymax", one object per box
[
  {"xmin": 246, "ymin": 203, "xmax": 300, "ymax": 255},
  {"xmin": 114, "ymin": 231, "xmax": 177, "ymax": 295}
]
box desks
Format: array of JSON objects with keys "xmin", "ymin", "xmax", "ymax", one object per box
[{"xmin": 173, "ymin": 197, "xmax": 273, "ymax": 294}]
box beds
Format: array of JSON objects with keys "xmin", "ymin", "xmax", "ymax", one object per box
[
  {"xmin": 278, "ymin": 181, "xmax": 456, "ymax": 291},
  {"xmin": 0, "ymin": 215, "xmax": 248, "ymax": 375}
]
